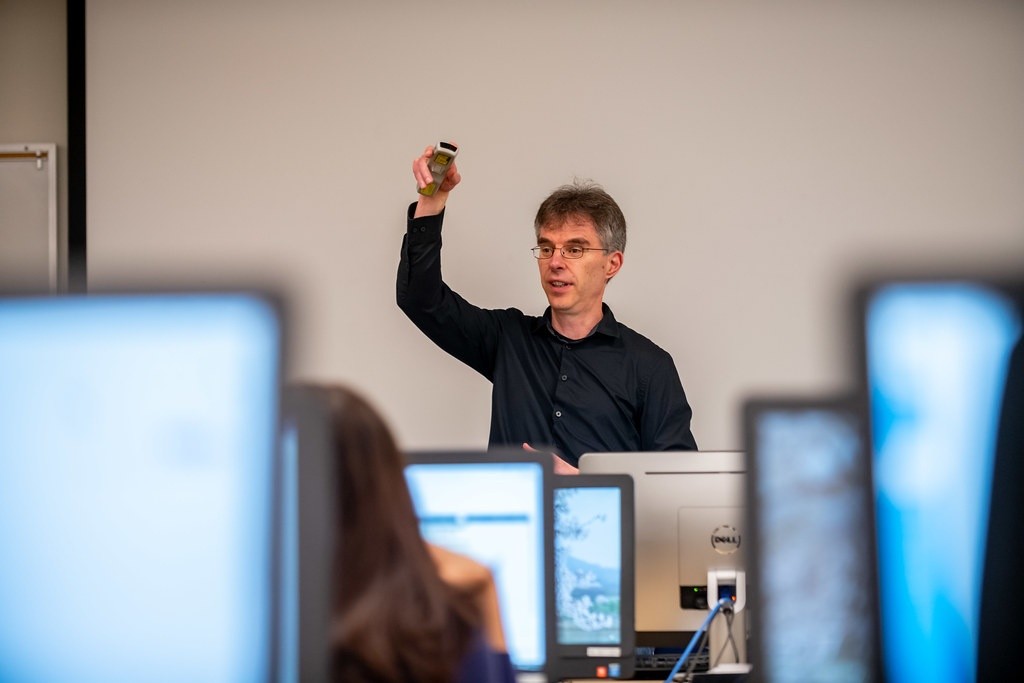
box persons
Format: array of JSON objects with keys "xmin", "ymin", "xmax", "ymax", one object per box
[
  {"xmin": 395, "ymin": 141, "xmax": 698, "ymax": 475},
  {"xmin": 279, "ymin": 377, "xmax": 517, "ymax": 683}
]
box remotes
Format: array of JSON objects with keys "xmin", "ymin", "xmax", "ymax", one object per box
[{"xmin": 416, "ymin": 141, "xmax": 458, "ymax": 197}]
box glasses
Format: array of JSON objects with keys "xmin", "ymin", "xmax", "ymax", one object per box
[{"xmin": 531, "ymin": 244, "xmax": 609, "ymax": 259}]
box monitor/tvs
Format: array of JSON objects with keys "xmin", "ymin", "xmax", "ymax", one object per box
[{"xmin": 0, "ymin": 275, "xmax": 1024, "ymax": 683}]
